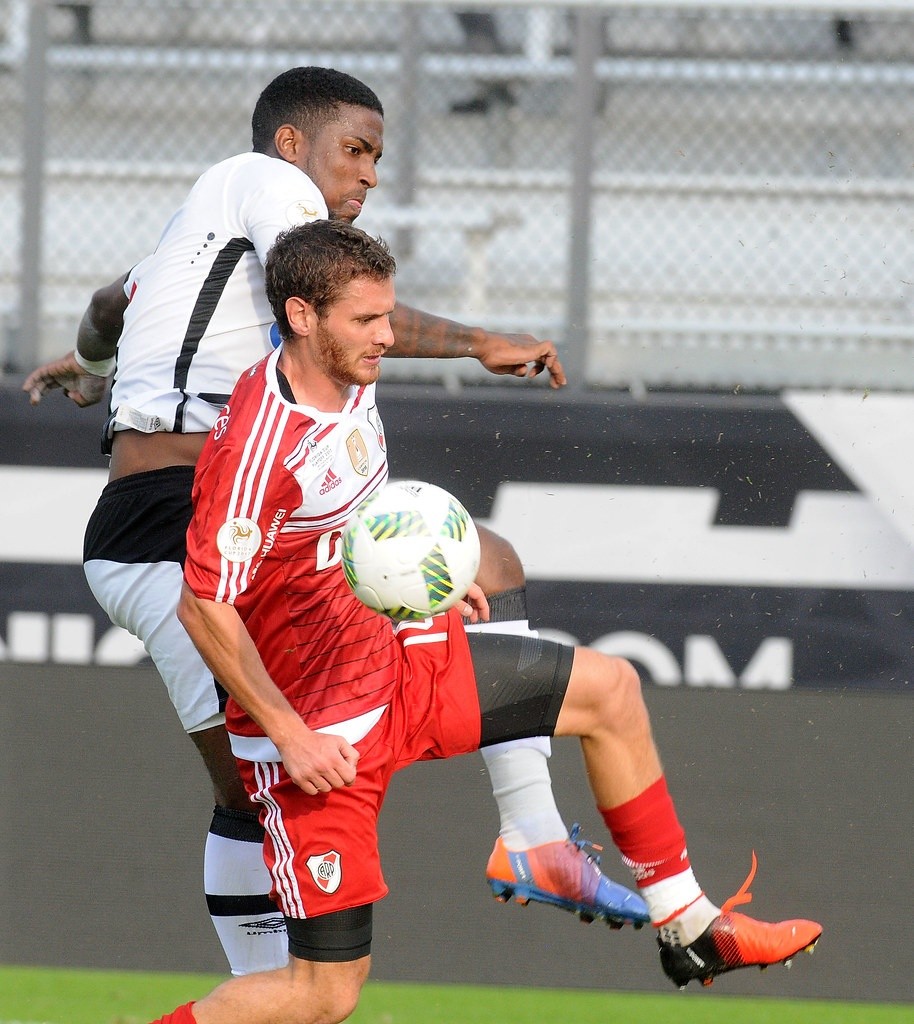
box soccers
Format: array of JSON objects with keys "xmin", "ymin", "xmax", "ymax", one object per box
[{"xmin": 340, "ymin": 475, "xmax": 481, "ymax": 622}]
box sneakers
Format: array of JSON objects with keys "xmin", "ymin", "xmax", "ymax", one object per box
[
  {"xmin": 485, "ymin": 823, "xmax": 651, "ymax": 931},
  {"xmin": 655, "ymin": 850, "xmax": 824, "ymax": 988}
]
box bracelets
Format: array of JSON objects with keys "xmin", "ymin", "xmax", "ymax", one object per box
[{"xmin": 75, "ymin": 350, "xmax": 115, "ymax": 377}]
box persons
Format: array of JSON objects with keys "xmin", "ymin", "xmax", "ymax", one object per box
[
  {"xmin": 150, "ymin": 218, "xmax": 823, "ymax": 1024},
  {"xmin": 23, "ymin": 66, "xmax": 651, "ymax": 978}
]
